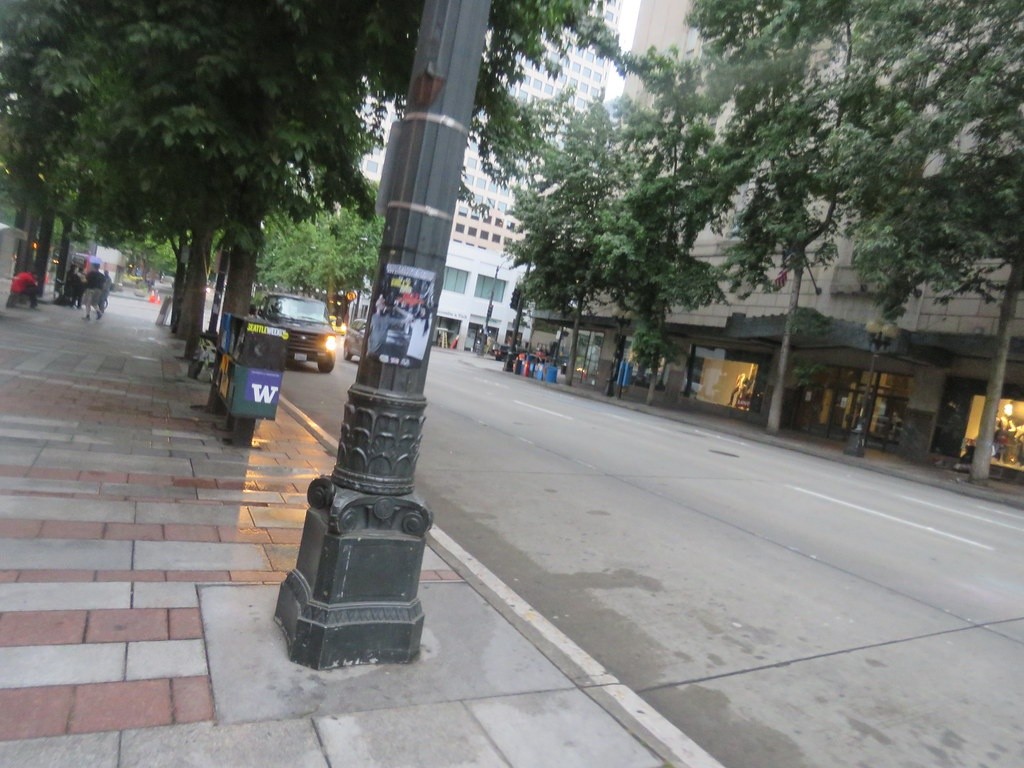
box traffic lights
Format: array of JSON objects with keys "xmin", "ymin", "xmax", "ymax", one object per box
[{"xmin": 487, "ymin": 304, "xmax": 492, "ymax": 317}]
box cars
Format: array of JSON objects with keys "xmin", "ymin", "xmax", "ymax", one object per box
[
  {"xmin": 329, "ymin": 316, "xmax": 346, "ymax": 336},
  {"xmin": 344, "ymin": 317, "xmax": 368, "ymax": 360},
  {"xmin": 124, "ymin": 274, "xmax": 143, "ymax": 285}
]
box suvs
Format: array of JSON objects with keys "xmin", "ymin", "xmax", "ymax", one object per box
[
  {"xmin": 257, "ymin": 294, "xmax": 337, "ymax": 372},
  {"xmin": 493, "ymin": 340, "xmax": 538, "ymax": 362},
  {"xmin": 533, "ymin": 342, "xmax": 569, "ymax": 368}
]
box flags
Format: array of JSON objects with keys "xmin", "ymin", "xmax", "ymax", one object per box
[{"xmin": 774, "ymin": 272, "xmax": 787, "ymax": 289}]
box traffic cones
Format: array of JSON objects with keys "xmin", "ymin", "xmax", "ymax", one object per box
[{"xmin": 148, "ymin": 289, "xmax": 161, "ymax": 305}]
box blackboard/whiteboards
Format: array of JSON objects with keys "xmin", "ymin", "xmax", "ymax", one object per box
[{"xmin": 475, "ymin": 344, "xmax": 484, "ymax": 356}]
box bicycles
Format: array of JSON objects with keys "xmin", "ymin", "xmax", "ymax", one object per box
[{"xmin": 74, "ymin": 290, "xmax": 108, "ymax": 310}]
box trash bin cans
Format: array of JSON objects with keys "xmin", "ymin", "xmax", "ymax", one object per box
[
  {"xmin": 214, "ymin": 312, "xmax": 288, "ymax": 422},
  {"xmin": 513, "ymin": 352, "xmax": 558, "ymax": 383},
  {"xmin": 160, "ymin": 271, "xmax": 166, "ymax": 284}
]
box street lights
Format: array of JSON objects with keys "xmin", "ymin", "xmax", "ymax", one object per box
[
  {"xmin": 605, "ymin": 306, "xmax": 635, "ymax": 398},
  {"xmin": 842, "ymin": 312, "xmax": 900, "ymax": 457},
  {"xmin": 480, "ymin": 259, "xmax": 516, "ymax": 344}
]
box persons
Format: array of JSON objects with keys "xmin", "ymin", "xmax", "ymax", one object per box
[
  {"xmin": 13, "ymin": 270, "xmax": 39, "ymax": 308},
  {"xmin": 70, "ymin": 268, "xmax": 87, "ymax": 309},
  {"xmin": 991, "ymin": 410, "xmax": 1017, "ymax": 456},
  {"xmin": 64, "ymin": 264, "xmax": 76, "ymax": 300},
  {"xmin": 367, "ymin": 293, "xmax": 432, "ymax": 361},
  {"xmin": 98, "ymin": 271, "xmax": 111, "ymax": 313},
  {"xmin": 81, "ymin": 264, "xmax": 103, "ymax": 320},
  {"xmin": 147, "ymin": 277, "xmax": 152, "ymax": 291},
  {"xmin": 728, "ymin": 367, "xmax": 756, "ymax": 406}
]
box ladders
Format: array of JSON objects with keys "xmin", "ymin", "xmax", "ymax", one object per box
[{"xmin": 438, "ymin": 329, "xmax": 448, "ymax": 349}]
